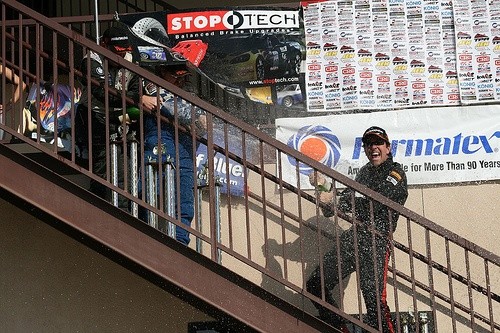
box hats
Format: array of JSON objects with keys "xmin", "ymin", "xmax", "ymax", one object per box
[{"xmin": 362, "ymin": 126, "xmax": 388, "ymax": 143}]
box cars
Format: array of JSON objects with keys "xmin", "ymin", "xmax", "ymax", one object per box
[{"xmin": 268, "ymin": 84, "xmax": 304, "ymax": 108}]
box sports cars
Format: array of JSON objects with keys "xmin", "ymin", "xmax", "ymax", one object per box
[{"xmin": 200, "ymin": 35, "xmax": 306, "ymax": 85}]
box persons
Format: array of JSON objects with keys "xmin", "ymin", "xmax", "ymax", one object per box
[
  {"xmin": 306, "ymin": 126, "xmax": 408, "ymax": 333},
  {"xmin": 73, "ymin": 18, "xmax": 202, "ymax": 245},
  {"xmin": 0, "ymin": 63, "xmax": 56, "ymax": 146}
]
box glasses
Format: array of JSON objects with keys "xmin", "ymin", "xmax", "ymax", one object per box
[{"xmin": 363, "ymin": 139, "xmax": 385, "ymax": 146}]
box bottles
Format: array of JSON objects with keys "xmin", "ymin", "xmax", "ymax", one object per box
[
  {"xmin": 317, "ymin": 181, "xmax": 334, "ymax": 218},
  {"xmin": 109, "ymin": 96, "xmax": 166, "ymax": 126}
]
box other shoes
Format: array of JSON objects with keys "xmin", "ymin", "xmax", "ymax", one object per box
[{"xmin": 316, "ymin": 316, "xmax": 346, "ymax": 330}]
